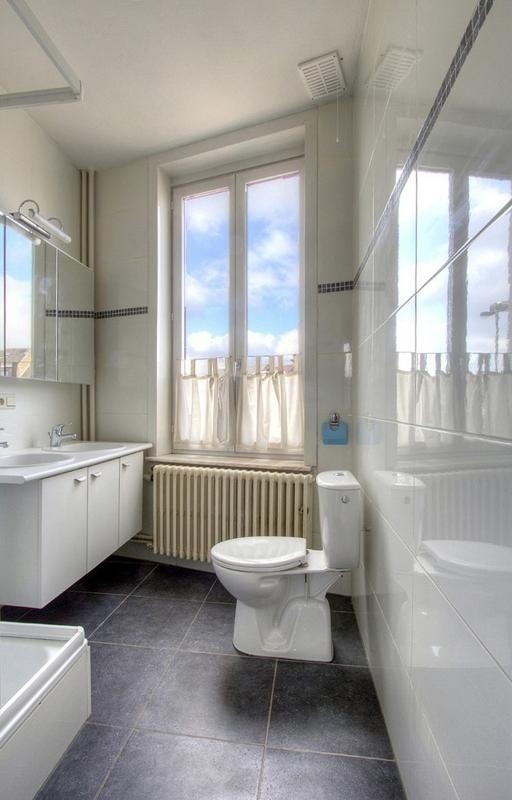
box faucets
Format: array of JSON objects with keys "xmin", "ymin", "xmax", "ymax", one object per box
[
  {"xmin": 51, "ymin": 423, "xmax": 78, "ymax": 446},
  {"xmin": 0, "ymin": 441, "xmax": 11, "ymax": 450}
]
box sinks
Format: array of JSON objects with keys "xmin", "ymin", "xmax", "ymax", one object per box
[
  {"xmin": 0, "ymin": 452, "xmax": 74, "ymax": 467},
  {"xmin": 44, "ymin": 440, "xmax": 127, "ymax": 452}
]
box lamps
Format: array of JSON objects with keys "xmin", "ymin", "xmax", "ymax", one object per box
[
  {"xmin": 10, "ymin": 200, "xmax": 73, "ymax": 245},
  {"xmin": 1, "ymin": 214, "xmax": 42, "ymax": 246}
]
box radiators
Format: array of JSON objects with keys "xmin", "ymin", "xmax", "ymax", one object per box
[
  {"xmin": 151, "ymin": 463, "xmax": 320, "ymax": 565},
  {"xmin": 394, "ymin": 450, "xmax": 509, "ymax": 584}
]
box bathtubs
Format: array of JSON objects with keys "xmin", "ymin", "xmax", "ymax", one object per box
[{"xmin": 2, "ymin": 619, "xmax": 91, "ymax": 799}]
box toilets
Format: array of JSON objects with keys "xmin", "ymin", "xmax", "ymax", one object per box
[
  {"xmin": 210, "ymin": 468, "xmax": 362, "ymax": 663},
  {"xmin": 367, "ymin": 469, "xmax": 510, "ymax": 669}
]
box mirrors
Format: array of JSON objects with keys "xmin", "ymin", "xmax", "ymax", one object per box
[{"xmin": 1, "ymin": 211, "xmax": 97, "ymax": 388}]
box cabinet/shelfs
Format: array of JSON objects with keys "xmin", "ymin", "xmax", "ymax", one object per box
[
  {"xmin": 1, "ymin": 458, "xmax": 120, "ymax": 610},
  {"xmin": 119, "ymin": 451, "xmax": 145, "ymax": 549}
]
